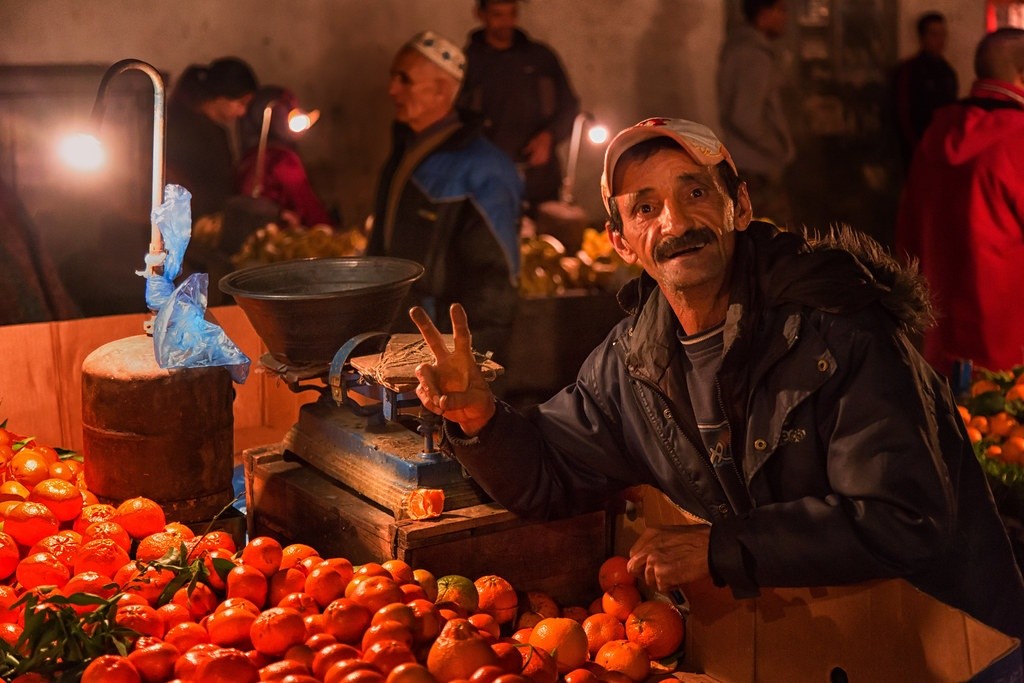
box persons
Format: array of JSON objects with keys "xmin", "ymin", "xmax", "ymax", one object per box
[
  {"xmin": 163, "ymin": 59, "xmax": 337, "ymax": 233},
  {"xmin": 456, "ymin": 0, "xmax": 580, "ymax": 221},
  {"xmin": 360, "ymin": 32, "xmax": 522, "ymax": 371},
  {"xmin": 717, "ymin": 0, "xmax": 795, "ymax": 235},
  {"xmin": 892, "ymin": 25, "xmax": 1024, "ymax": 380},
  {"xmin": 408, "ymin": 117, "xmax": 1024, "ymax": 683},
  {"xmin": 883, "ymin": 12, "xmax": 959, "ymax": 175}
]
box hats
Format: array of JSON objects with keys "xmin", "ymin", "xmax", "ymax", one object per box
[
  {"xmin": 407, "ymin": 32, "xmax": 465, "ymax": 84},
  {"xmin": 601, "ymin": 116, "xmax": 739, "ymax": 215}
]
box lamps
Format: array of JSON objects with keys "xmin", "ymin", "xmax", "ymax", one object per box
[
  {"xmin": 58, "ymin": 58, "xmax": 167, "ymax": 336},
  {"xmin": 563, "ymin": 112, "xmax": 606, "ymax": 203},
  {"xmin": 251, "ymin": 90, "xmax": 320, "ymax": 191}
]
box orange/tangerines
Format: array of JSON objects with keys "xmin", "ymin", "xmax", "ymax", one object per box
[
  {"xmin": 954, "ymin": 372, "xmax": 1024, "ymax": 470},
  {"xmin": 0, "ymin": 427, "xmax": 682, "ymax": 683}
]
